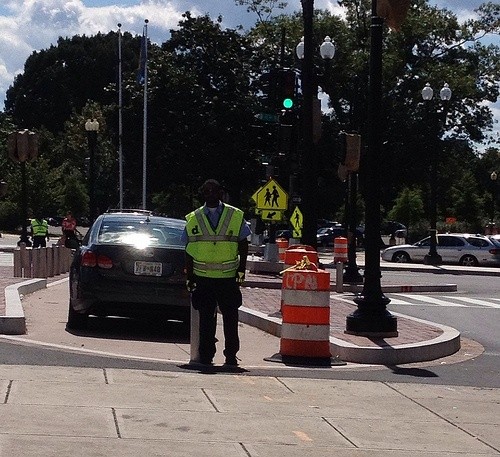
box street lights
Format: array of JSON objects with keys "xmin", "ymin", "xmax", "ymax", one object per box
[
  {"xmin": 421, "ymin": 82, "xmax": 452, "ymax": 265},
  {"xmin": 7, "ymin": 128, "xmax": 36, "ymax": 247},
  {"xmin": 85, "ymin": 118, "xmax": 99, "ymax": 228},
  {"xmin": 490, "ymin": 171, "xmax": 498, "ymax": 235}
]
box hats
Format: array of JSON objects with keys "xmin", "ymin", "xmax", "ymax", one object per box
[{"xmin": 66, "ymin": 211, "xmax": 72, "ymax": 215}]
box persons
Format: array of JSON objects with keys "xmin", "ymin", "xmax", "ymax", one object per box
[
  {"xmin": 30, "ymin": 211, "xmax": 49, "ymax": 248},
  {"xmin": 181, "ymin": 179, "xmax": 253, "ymax": 365},
  {"xmin": 61, "ymin": 210, "xmax": 76, "ymax": 239}
]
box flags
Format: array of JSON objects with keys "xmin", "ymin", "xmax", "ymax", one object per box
[{"xmin": 137, "ymin": 34, "xmax": 146, "ymax": 83}]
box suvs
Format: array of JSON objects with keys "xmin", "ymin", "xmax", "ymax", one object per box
[
  {"xmin": 316, "ymin": 227, "xmax": 345, "ymax": 245},
  {"xmin": 380, "ymin": 231, "xmax": 500, "ymax": 268}
]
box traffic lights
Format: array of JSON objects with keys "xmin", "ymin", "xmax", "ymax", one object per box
[{"xmin": 277, "ymin": 70, "xmax": 295, "ymax": 128}]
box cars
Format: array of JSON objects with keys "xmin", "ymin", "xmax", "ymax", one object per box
[
  {"xmin": 275, "ymin": 229, "xmax": 292, "ymax": 242},
  {"xmin": 64, "ymin": 213, "xmax": 191, "ymax": 338},
  {"xmin": 379, "ymin": 221, "xmax": 408, "ymax": 238}
]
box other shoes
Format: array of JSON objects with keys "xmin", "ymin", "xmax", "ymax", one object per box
[
  {"xmin": 226, "ymin": 356, "xmax": 242, "ymax": 364},
  {"xmin": 189, "ymin": 357, "xmax": 214, "ymax": 367}
]
